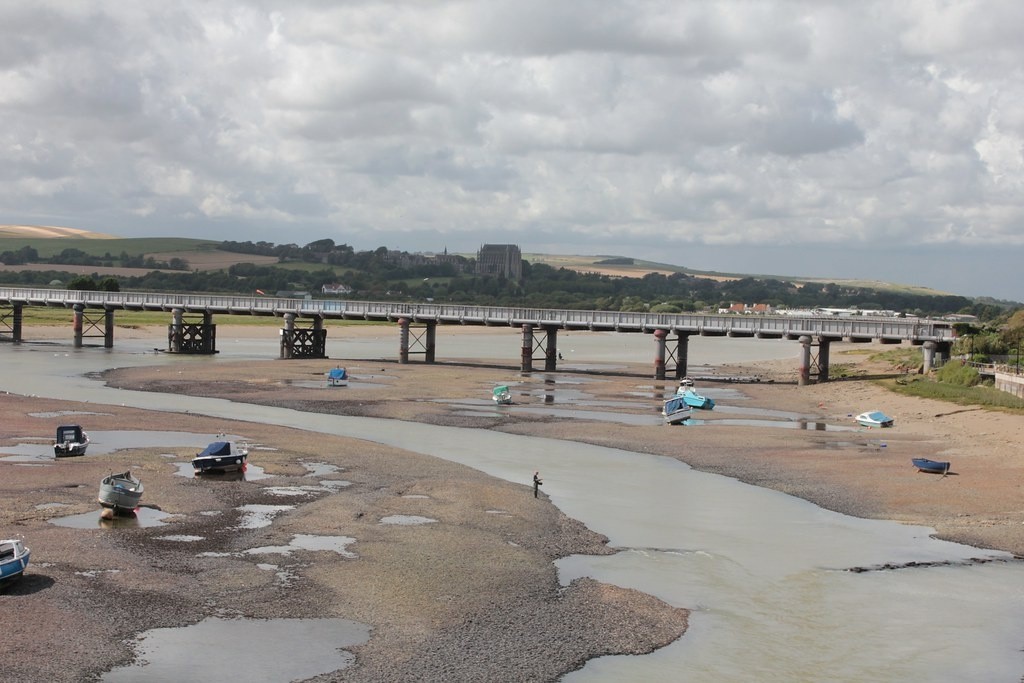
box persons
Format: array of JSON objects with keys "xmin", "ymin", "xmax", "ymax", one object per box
[{"xmin": 533, "ymin": 471, "xmax": 543, "ymax": 499}]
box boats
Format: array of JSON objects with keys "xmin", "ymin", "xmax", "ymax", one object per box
[
  {"xmin": 912, "ymin": 457, "xmax": 951, "ymax": 474},
  {"xmin": 98, "ymin": 469, "xmax": 145, "ymax": 521},
  {"xmin": 191, "ymin": 440, "xmax": 248, "ymax": 473},
  {"xmin": 671, "ymin": 385, "xmax": 716, "ymax": 410},
  {"xmin": 854, "ymin": 411, "xmax": 894, "ymax": 429},
  {"xmin": 492, "ymin": 385, "xmax": 512, "ymax": 404},
  {"xmin": 53, "ymin": 422, "xmax": 90, "ymax": 456},
  {"xmin": 680, "ymin": 377, "xmax": 694, "ymax": 387},
  {"xmin": 662, "ymin": 395, "xmax": 692, "ymax": 426},
  {"xmin": 327, "ymin": 367, "xmax": 349, "ymax": 386},
  {"xmin": 0, "ymin": 535, "xmax": 31, "ymax": 591}
]
